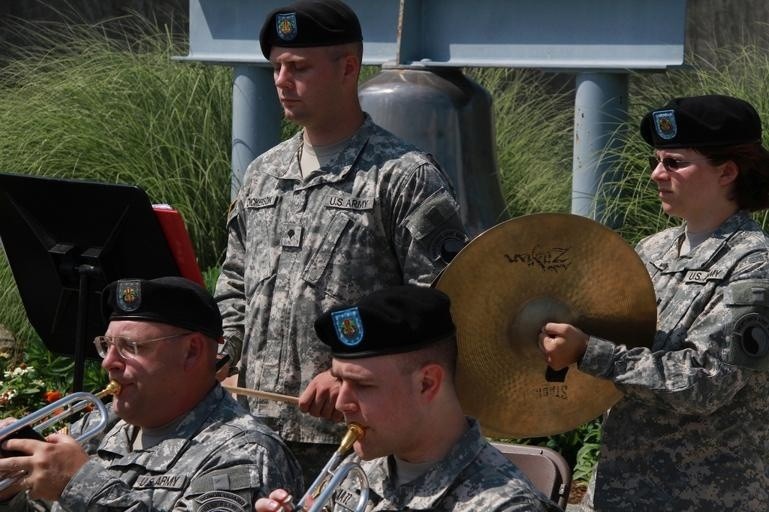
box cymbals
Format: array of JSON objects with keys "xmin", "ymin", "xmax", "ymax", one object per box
[{"xmin": 430, "ymin": 213, "xmax": 657, "ymax": 439}]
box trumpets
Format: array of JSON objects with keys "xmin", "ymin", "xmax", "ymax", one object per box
[
  {"xmin": 0, "ymin": 380, "xmax": 121, "ymax": 491},
  {"xmin": 280, "ymin": 425, "xmax": 369, "ymax": 512}
]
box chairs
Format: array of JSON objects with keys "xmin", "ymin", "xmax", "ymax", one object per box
[{"xmin": 483, "ymin": 441, "xmax": 573, "ymax": 511}]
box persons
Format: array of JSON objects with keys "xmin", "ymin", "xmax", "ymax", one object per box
[
  {"xmin": 1, "ymin": 278, "xmax": 303, "ymax": 512},
  {"xmin": 529, "ymin": 94, "xmax": 768, "ymax": 511},
  {"xmin": 188, "ymin": 0, "xmax": 468, "ymax": 493},
  {"xmin": 252, "ymin": 283, "xmax": 563, "ymax": 512}
]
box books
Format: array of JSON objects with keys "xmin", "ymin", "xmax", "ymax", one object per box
[{"xmin": 152, "ymin": 198, "xmax": 204, "ymax": 289}]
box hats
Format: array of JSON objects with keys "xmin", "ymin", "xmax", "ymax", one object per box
[
  {"xmin": 312, "ymin": 286, "xmax": 454, "ymax": 362},
  {"xmin": 258, "ymin": 0, "xmax": 363, "ymax": 62},
  {"xmin": 640, "ymin": 95, "xmax": 763, "ymax": 150},
  {"xmin": 99, "ymin": 276, "xmax": 224, "ymax": 342}
]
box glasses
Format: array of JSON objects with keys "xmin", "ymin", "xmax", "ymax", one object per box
[
  {"xmin": 93, "ymin": 331, "xmax": 192, "ymax": 362},
  {"xmin": 647, "ymin": 155, "xmax": 717, "ymax": 170}
]
